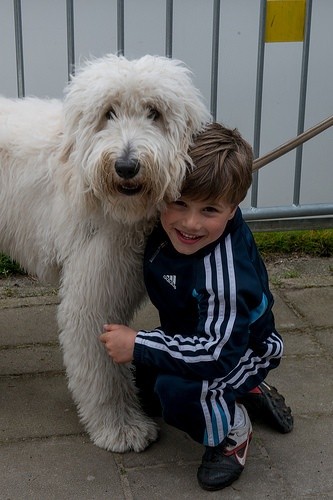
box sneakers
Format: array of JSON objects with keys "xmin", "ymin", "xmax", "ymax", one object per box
[
  {"xmin": 197, "ymin": 404, "xmax": 253, "ymax": 492},
  {"xmin": 235, "ymin": 381, "xmax": 294, "ymax": 434}
]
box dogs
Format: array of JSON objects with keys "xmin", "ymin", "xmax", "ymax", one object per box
[{"xmin": 0, "ymin": 48, "xmax": 214, "ymax": 453}]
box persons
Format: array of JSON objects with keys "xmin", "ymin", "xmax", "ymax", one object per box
[{"xmin": 98, "ymin": 124, "xmax": 293, "ymax": 491}]
